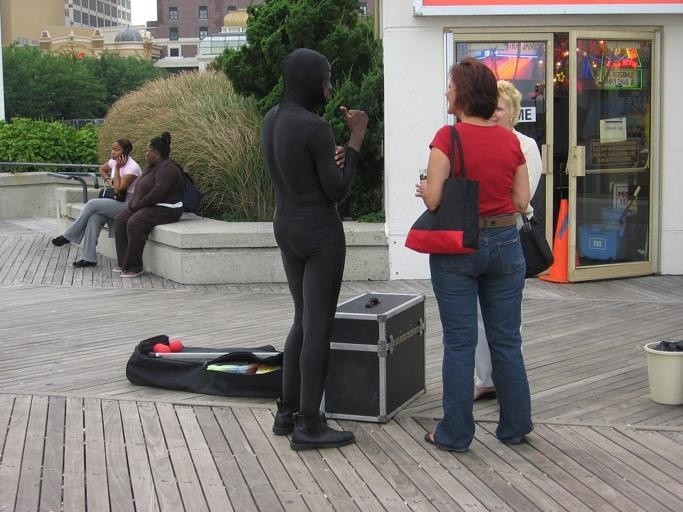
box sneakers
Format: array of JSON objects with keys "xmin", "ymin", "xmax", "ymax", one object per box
[
  {"xmin": 474, "ymin": 383, "xmax": 496, "ymax": 401},
  {"xmin": 72, "ymin": 259, "xmax": 97, "ymax": 267},
  {"xmin": 51, "ymin": 235, "xmax": 70, "ymax": 246}
]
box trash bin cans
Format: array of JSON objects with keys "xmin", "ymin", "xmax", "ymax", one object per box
[{"xmin": 643, "ymin": 340, "xmax": 683, "ymax": 406}]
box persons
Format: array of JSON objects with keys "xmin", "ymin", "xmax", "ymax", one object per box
[
  {"xmin": 51, "ymin": 139, "xmax": 143, "ymax": 269},
  {"xmin": 415, "ymin": 57, "xmax": 534, "ymax": 453},
  {"xmin": 472, "ymin": 80, "xmax": 543, "ymax": 402},
  {"xmin": 261, "ymin": 47, "xmax": 368, "ymax": 452},
  {"xmin": 112, "ymin": 131, "xmax": 185, "ymax": 278}
]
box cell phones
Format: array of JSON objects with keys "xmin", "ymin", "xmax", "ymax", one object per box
[{"xmin": 121, "ymin": 149, "xmax": 128, "ymax": 156}]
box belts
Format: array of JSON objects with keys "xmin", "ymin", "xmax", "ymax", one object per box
[{"xmin": 478, "ymin": 213, "xmax": 517, "ymax": 229}]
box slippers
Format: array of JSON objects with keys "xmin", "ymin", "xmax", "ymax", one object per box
[{"xmin": 120, "ymin": 269, "xmax": 145, "ymax": 278}]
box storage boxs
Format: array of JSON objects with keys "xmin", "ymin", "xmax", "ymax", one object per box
[{"xmin": 322, "ymin": 293, "xmax": 426, "ymax": 424}]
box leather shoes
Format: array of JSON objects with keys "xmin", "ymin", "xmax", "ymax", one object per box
[{"xmin": 113, "ymin": 267, "xmax": 124, "ymax": 273}]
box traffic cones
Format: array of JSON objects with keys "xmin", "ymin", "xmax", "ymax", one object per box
[{"xmin": 538, "ymin": 197, "xmax": 580, "ymax": 284}]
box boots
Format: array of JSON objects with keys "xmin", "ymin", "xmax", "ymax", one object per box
[
  {"xmin": 290, "ymin": 410, "xmax": 356, "ymax": 451},
  {"xmin": 272, "ymin": 397, "xmax": 327, "ymax": 435}
]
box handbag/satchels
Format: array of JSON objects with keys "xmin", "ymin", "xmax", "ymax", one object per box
[
  {"xmin": 519, "ymin": 215, "xmax": 555, "ymax": 279},
  {"xmin": 98, "ymin": 188, "xmax": 127, "ymax": 202},
  {"xmin": 404, "ymin": 177, "xmax": 480, "ymax": 254}
]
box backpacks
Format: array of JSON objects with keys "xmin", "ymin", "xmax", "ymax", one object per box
[{"xmin": 182, "ymin": 172, "xmax": 201, "ymax": 213}]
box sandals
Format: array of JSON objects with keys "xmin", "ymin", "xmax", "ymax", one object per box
[{"xmin": 424, "ymin": 432, "xmax": 435, "ymax": 444}]
